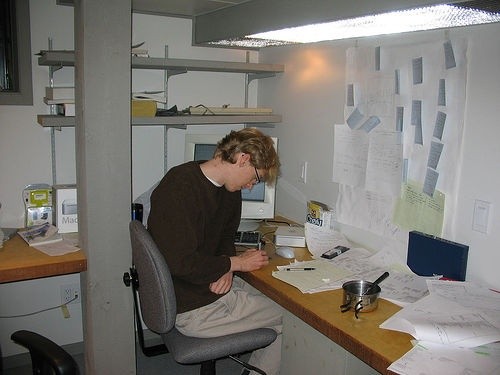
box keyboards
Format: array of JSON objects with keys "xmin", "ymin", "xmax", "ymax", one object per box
[{"xmin": 234, "ymin": 231, "xmax": 260, "ymax": 246}]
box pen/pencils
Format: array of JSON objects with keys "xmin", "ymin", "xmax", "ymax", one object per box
[
  {"xmin": 287, "ymin": 268, "xmax": 316, "ymax": 270},
  {"xmin": 258, "ymin": 241, "xmax": 261, "ymax": 250}
]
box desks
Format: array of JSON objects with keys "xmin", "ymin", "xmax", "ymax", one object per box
[
  {"xmin": 232, "ymin": 216, "xmax": 417, "ymax": 375},
  {"xmin": 0, "ymin": 236, "xmax": 88, "ymax": 285}
]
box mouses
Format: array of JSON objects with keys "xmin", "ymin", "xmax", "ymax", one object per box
[{"xmin": 276, "ymin": 247, "xmax": 295, "ymax": 258}]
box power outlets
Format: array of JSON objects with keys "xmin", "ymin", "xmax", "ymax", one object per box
[{"xmin": 60, "ymin": 285, "xmax": 81, "ymax": 306}]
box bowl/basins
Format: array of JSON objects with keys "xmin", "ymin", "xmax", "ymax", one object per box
[{"xmin": 341, "ymin": 279, "xmax": 381, "ymax": 313}]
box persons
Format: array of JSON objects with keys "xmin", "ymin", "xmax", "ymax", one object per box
[{"xmin": 147, "ymin": 128, "xmax": 282, "ymax": 375}]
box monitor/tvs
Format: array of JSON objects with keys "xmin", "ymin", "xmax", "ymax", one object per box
[{"xmin": 184, "ymin": 134, "xmax": 278, "ymax": 232}]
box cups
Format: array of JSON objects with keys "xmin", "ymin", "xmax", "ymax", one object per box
[{"xmin": 131, "ymin": 203, "xmax": 143, "ymax": 222}]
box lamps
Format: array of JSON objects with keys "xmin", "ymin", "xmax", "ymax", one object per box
[{"xmin": 193, "ymin": 0, "xmax": 500, "ymax": 50}]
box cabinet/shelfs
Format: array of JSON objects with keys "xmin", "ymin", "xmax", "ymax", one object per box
[{"xmin": 38, "ymin": 39, "xmax": 286, "ymax": 184}]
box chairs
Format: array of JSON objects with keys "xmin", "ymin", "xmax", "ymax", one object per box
[
  {"xmin": 130, "ymin": 218, "xmax": 277, "ymax": 375},
  {"xmin": 10, "ymin": 329, "xmax": 80, "ymax": 375}
]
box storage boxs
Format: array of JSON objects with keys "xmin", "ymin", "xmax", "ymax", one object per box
[
  {"xmin": 131, "ymin": 100, "xmax": 157, "ymax": 117},
  {"xmin": 306, "ymin": 200, "xmax": 331, "ymax": 228},
  {"xmin": 22, "ymin": 185, "xmax": 78, "ymax": 234}
]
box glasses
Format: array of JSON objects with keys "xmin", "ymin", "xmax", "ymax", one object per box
[{"xmin": 253, "ymin": 168, "xmax": 260, "ymax": 185}]
